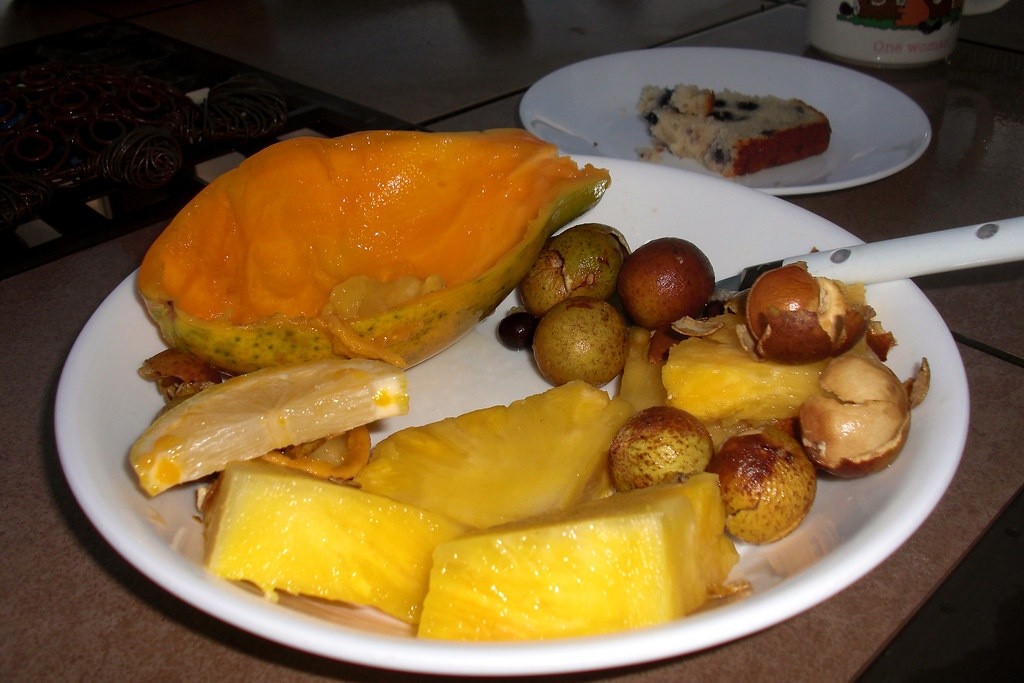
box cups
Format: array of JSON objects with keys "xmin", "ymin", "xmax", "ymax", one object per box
[{"xmin": 804, "ymin": 0, "xmax": 1012, "ymax": 68}]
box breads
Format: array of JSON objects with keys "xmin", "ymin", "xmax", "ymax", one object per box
[{"xmin": 633, "ymin": 82, "xmax": 831, "ymax": 175}]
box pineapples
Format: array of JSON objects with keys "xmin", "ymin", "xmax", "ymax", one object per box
[{"xmin": 200, "ymin": 339, "xmax": 832, "ymax": 640}]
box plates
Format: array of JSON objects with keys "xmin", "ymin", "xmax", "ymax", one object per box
[
  {"xmin": 519, "ymin": 46, "xmax": 931, "ymax": 197},
  {"xmin": 54, "ymin": 152, "xmax": 973, "ymax": 677}
]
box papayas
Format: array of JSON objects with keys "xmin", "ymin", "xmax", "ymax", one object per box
[{"xmin": 136, "ymin": 119, "xmax": 611, "ymax": 369}]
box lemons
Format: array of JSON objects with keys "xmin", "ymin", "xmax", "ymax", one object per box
[{"xmin": 121, "ymin": 360, "xmax": 406, "ymax": 494}]
